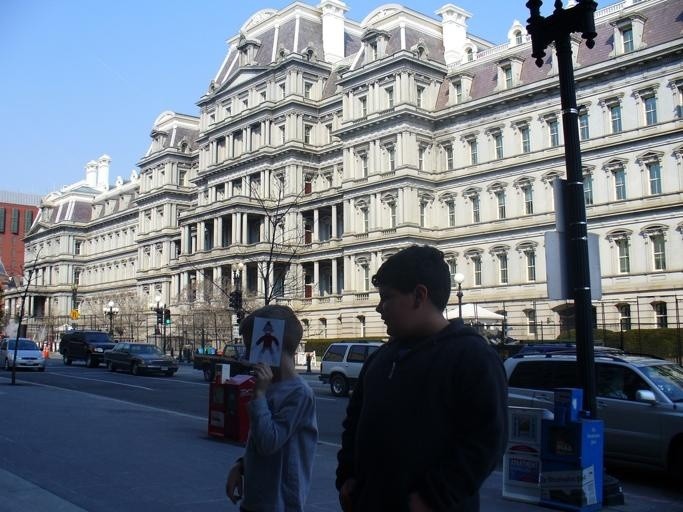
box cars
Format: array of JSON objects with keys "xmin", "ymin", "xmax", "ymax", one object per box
[
  {"xmin": 1, "ymin": 338, "xmax": 45, "ymax": 373},
  {"xmin": 105, "ymin": 342, "xmax": 178, "ymax": 377}
]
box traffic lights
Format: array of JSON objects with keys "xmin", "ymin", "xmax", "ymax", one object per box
[
  {"xmin": 228, "ymin": 292, "xmax": 237, "ymax": 308},
  {"xmin": 165, "ymin": 309, "xmax": 170, "ymax": 324},
  {"xmin": 156, "ymin": 309, "xmax": 163, "ymax": 323}
]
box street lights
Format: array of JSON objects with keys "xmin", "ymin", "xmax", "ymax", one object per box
[
  {"xmin": 103, "ymin": 300, "xmax": 119, "ymax": 335},
  {"xmin": 150, "ymin": 296, "xmax": 164, "ymax": 335},
  {"xmin": 453, "ymin": 273, "xmax": 465, "ymax": 318}
]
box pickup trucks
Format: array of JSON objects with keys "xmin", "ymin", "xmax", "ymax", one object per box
[{"xmin": 194, "ymin": 343, "xmax": 247, "ymax": 381}]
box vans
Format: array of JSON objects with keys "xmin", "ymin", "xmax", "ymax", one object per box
[
  {"xmin": 502, "ymin": 342, "xmax": 682, "ymax": 487},
  {"xmin": 60, "ymin": 328, "xmax": 118, "ymax": 367},
  {"xmin": 319, "ymin": 338, "xmax": 386, "ymax": 394}
]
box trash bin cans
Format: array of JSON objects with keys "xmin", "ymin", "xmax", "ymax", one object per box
[
  {"xmin": 501, "ymin": 386, "xmax": 607, "ymax": 512},
  {"xmin": 207, "ymin": 362, "xmax": 257, "ymax": 448}
]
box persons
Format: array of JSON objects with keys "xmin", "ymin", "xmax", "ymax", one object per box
[
  {"xmin": 255, "ymin": 322, "xmax": 280, "ymax": 364},
  {"xmin": 336, "ymin": 247, "xmax": 509, "ymax": 512},
  {"xmin": 605, "ymin": 376, "xmax": 628, "ymax": 400},
  {"xmin": 227, "ymin": 305, "xmax": 318, "ymax": 512}
]
